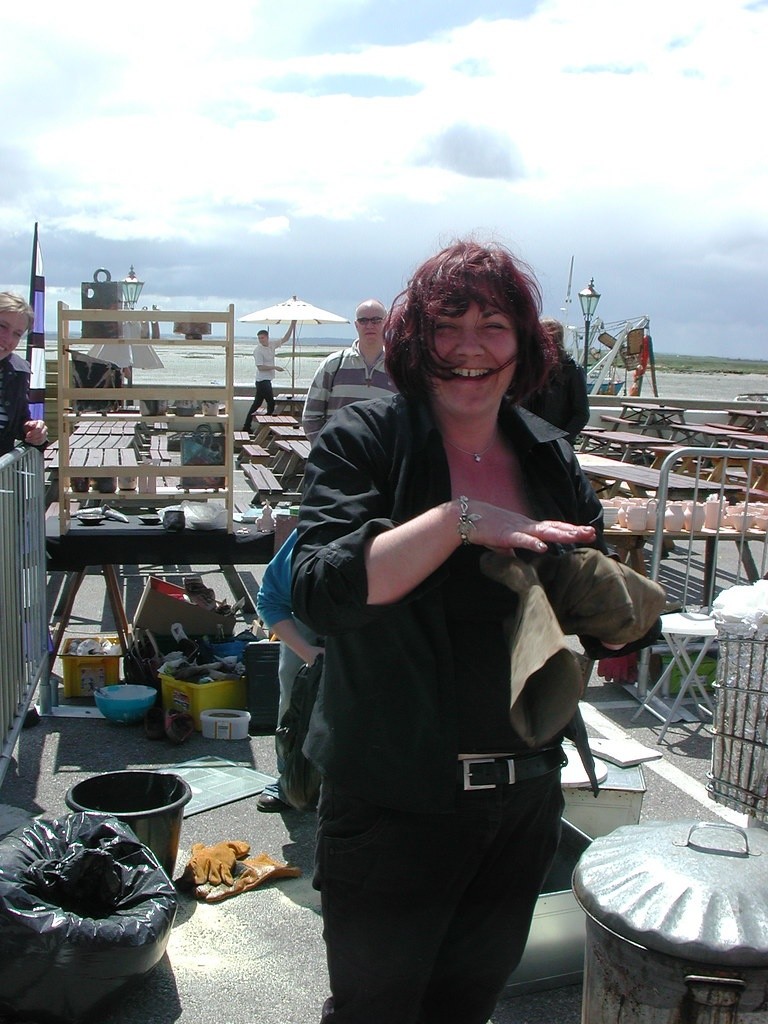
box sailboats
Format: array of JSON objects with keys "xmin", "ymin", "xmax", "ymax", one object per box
[{"xmin": 558, "ymin": 255, "xmax": 626, "ymax": 396}]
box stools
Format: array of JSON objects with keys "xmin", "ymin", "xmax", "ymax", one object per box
[{"xmin": 630, "ymin": 606, "xmax": 721, "ymax": 742}]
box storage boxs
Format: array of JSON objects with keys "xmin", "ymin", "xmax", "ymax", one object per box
[
  {"xmin": 563, "ymin": 740, "xmax": 653, "ymax": 836},
  {"xmin": 496, "ymin": 816, "xmax": 593, "ymax": 998},
  {"xmin": 61, "ymin": 635, "xmax": 122, "ymax": 698},
  {"xmin": 131, "ymin": 576, "xmax": 235, "ymax": 645},
  {"xmin": 157, "ymin": 671, "xmax": 251, "ymax": 732}
]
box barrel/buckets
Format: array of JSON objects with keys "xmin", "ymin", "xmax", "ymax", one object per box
[
  {"xmin": 65, "ymin": 770, "xmax": 192, "ymax": 883},
  {"xmin": 572, "ymin": 819, "xmax": 768, "ymax": 1024},
  {"xmin": 242, "ymin": 641, "xmax": 281, "ymax": 731}
]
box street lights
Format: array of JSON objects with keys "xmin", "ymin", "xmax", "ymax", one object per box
[
  {"xmin": 120, "ymin": 265, "xmax": 144, "ymax": 406},
  {"xmin": 578, "ymin": 278, "xmax": 601, "ymax": 391}
]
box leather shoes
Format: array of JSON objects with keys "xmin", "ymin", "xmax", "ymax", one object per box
[{"xmin": 257, "ymin": 793, "xmax": 289, "ymax": 812}]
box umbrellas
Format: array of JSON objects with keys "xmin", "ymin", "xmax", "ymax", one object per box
[{"xmin": 237, "ymin": 297, "xmax": 351, "ymax": 416}]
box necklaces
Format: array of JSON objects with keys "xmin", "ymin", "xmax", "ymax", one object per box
[{"xmin": 442, "ymin": 432, "xmax": 497, "ymax": 462}]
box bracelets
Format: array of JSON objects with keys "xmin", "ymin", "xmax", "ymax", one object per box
[{"xmin": 458, "ymin": 495, "xmax": 481, "ymax": 547}]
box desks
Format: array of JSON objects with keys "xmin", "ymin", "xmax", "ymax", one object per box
[
  {"xmin": 239, "ymin": 425, "xmax": 304, "ymax": 474},
  {"xmin": 596, "ymin": 518, "xmax": 768, "ymax": 586},
  {"xmin": 53, "ymin": 435, "xmax": 135, "ymax": 449},
  {"xmin": 74, "ymin": 426, "xmax": 142, "ymax": 459},
  {"xmin": 610, "ymin": 402, "xmax": 692, "ymax": 446},
  {"xmin": 74, "ymin": 421, "xmax": 143, "ymax": 449},
  {"xmin": 237, "ymin": 413, "xmax": 300, "ymax": 462},
  {"xmin": 574, "ymin": 454, "xmax": 758, "ymax": 582},
  {"xmin": 712, "ymin": 409, "xmax": 768, "ymax": 447},
  {"xmin": 250, "ymin": 438, "xmax": 312, "ymax": 505},
  {"xmin": 579, "ymin": 430, "xmax": 675, "ymax": 497},
  {"xmin": 671, "ymin": 421, "xmax": 748, "ymax": 450},
  {"xmin": 582, "ymin": 425, "xmax": 606, "ymax": 431},
  {"xmin": 43, "ymin": 449, "xmax": 135, "ymax": 502},
  {"xmin": 712, "ymin": 435, "xmax": 768, "ymax": 485},
  {"xmin": 628, "ymin": 444, "xmax": 752, "ymax": 495},
  {"xmin": 252, "ymin": 393, "xmax": 308, "ymax": 435},
  {"xmin": 38, "ymin": 510, "xmax": 278, "ymax": 681}
]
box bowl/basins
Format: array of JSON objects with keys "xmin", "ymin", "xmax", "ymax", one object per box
[
  {"xmin": 94, "ymin": 685, "xmax": 158, "ymax": 725},
  {"xmin": 201, "ymin": 403, "xmax": 219, "ymax": 416},
  {"xmin": 137, "ymin": 514, "xmax": 161, "ymax": 525},
  {"xmin": 69, "ymin": 476, "xmax": 137, "ymax": 493},
  {"xmin": 140, "ymin": 400, "xmax": 169, "ymax": 416},
  {"xmin": 725, "ymin": 502, "xmax": 768, "ymax": 532},
  {"xmin": 77, "ymin": 513, "xmax": 106, "ymax": 525},
  {"xmin": 602, "ymin": 507, "xmax": 619, "ymax": 528}
]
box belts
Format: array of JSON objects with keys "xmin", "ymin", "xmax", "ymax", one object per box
[{"xmin": 457, "ymin": 746, "xmax": 568, "ymax": 791}]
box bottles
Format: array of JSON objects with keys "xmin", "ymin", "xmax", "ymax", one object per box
[
  {"xmin": 612, "ymin": 494, "xmax": 705, "ymax": 532},
  {"xmin": 213, "ymin": 624, "xmax": 226, "ymax": 644},
  {"xmin": 162, "ymin": 510, "xmax": 185, "ymax": 531}
]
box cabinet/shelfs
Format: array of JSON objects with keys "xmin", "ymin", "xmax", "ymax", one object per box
[{"xmin": 53, "ymin": 300, "xmax": 236, "ymax": 535}]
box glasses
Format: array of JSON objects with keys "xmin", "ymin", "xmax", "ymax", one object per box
[{"xmin": 356, "ymin": 316, "xmax": 386, "ymax": 325}]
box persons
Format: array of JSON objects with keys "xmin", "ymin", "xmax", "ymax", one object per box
[
  {"xmin": 520, "ymin": 317, "xmax": 590, "ymax": 449},
  {"xmin": 302, "ymin": 297, "xmax": 404, "ymax": 454},
  {"xmin": 242, "ymin": 321, "xmax": 298, "ymax": 434},
  {"xmin": 258, "ymin": 526, "xmax": 325, "ymax": 814},
  {"xmin": 0, "ymin": 292, "xmax": 48, "ymax": 728},
  {"xmin": 290, "ymin": 235, "xmax": 668, "ymax": 1024}
]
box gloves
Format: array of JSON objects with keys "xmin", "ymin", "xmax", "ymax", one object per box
[
  {"xmin": 175, "ymin": 852, "xmax": 302, "ymax": 903},
  {"xmin": 479, "ymin": 541, "xmax": 584, "ymax": 749},
  {"xmin": 543, "ymin": 547, "xmax": 666, "ymax": 645},
  {"xmin": 183, "ymin": 840, "xmax": 251, "ymax": 887}
]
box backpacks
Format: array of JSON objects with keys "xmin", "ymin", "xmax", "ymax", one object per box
[{"xmin": 275, "ymin": 653, "xmax": 323, "ymax": 813}]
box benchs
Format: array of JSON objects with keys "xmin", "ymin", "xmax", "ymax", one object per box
[{"xmin": 35, "ymin": 400, "xmax": 768, "ymax": 528}]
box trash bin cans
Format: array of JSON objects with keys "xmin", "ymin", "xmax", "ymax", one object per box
[{"xmin": 573, "ymin": 820, "xmax": 767, "ymax": 1024}]
